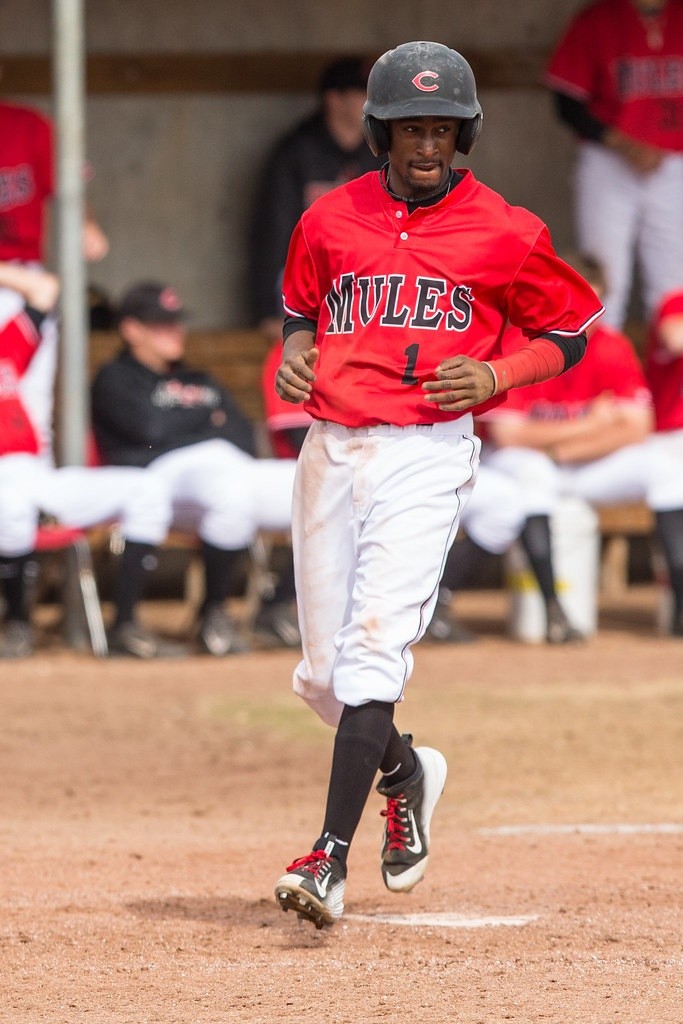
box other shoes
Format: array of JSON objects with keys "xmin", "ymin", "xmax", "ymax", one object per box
[
  {"xmin": 547, "ymin": 601, "xmax": 586, "ymax": 645},
  {"xmin": 428, "ymin": 586, "xmax": 454, "ymax": 639},
  {"xmin": 259, "ymin": 604, "xmax": 300, "ymax": 647},
  {"xmin": 202, "ymin": 600, "xmax": 240, "ymax": 655},
  {"xmin": 107, "ymin": 620, "xmax": 158, "ymax": 659},
  {"xmin": 4, "ymin": 621, "xmax": 35, "ymax": 657}
]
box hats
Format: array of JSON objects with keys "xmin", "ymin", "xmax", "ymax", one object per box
[{"xmin": 119, "ymin": 281, "xmax": 188, "ymax": 323}]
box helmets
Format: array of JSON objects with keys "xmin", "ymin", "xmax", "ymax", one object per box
[{"xmin": 360, "ymin": 41, "xmax": 484, "ymax": 159}]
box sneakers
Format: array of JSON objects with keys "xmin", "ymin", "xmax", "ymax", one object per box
[
  {"xmin": 274, "ymin": 832, "xmax": 349, "ymax": 930},
  {"xmin": 376, "ymin": 734, "xmax": 447, "ymax": 892}
]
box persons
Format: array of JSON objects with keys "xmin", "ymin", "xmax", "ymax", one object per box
[
  {"xmin": 275, "ymin": 41, "xmax": 605, "ymax": 930},
  {"xmin": 0, "ymin": 0, "xmax": 683, "ymax": 648}
]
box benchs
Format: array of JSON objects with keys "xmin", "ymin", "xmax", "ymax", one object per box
[{"xmin": 85, "ymin": 331, "xmax": 650, "ymax": 547}]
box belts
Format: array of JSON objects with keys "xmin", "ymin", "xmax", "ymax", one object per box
[{"xmin": 381, "ymin": 422, "xmax": 433, "ymax": 427}]
